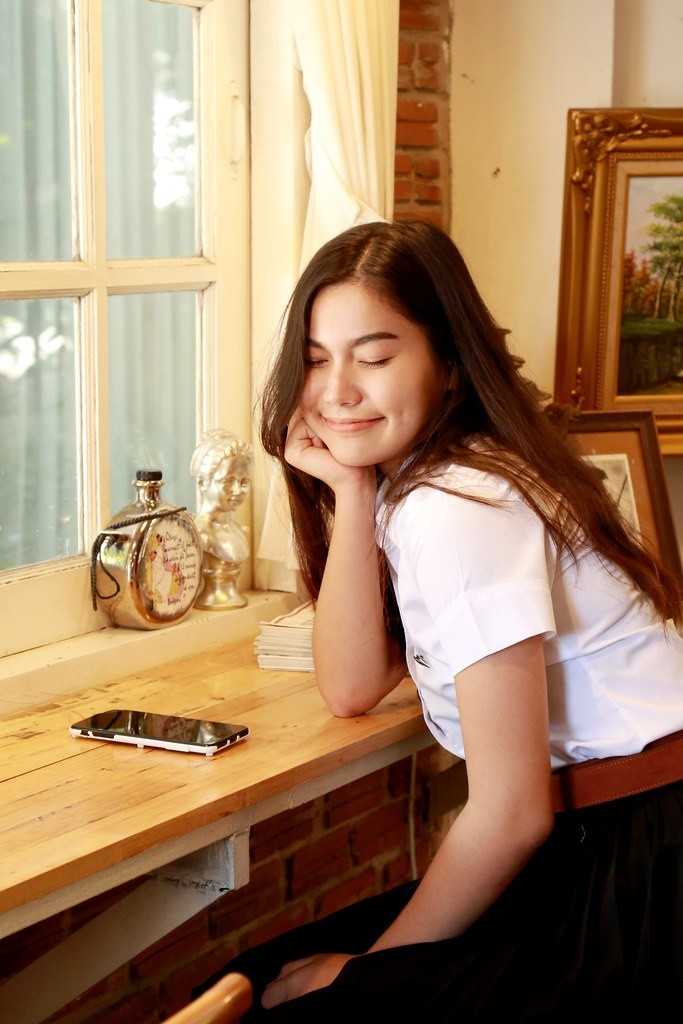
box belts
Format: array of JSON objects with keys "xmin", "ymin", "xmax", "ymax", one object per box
[{"xmin": 550, "ymin": 729, "xmax": 683, "ymax": 813}]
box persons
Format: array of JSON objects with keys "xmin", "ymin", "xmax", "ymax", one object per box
[
  {"xmin": 189, "ymin": 429, "xmax": 253, "ymax": 610},
  {"xmin": 237, "ymin": 221, "xmax": 683, "ymax": 1024}
]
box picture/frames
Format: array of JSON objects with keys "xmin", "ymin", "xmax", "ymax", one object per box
[
  {"xmin": 546, "ymin": 408, "xmax": 683, "ymax": 594},
  {"xmin": 552, "ymin": 107, "xmax": 683, "ymax": 455}
]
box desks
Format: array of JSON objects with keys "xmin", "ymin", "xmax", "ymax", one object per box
[{"xmin": 0, "ymin": 632, "xmax": 468, "ymax": 1024}]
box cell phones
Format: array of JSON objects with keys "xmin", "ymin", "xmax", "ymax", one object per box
[{"xmin": 68, "ymin": 708, "xmax": 249, "ymax": 757}]
